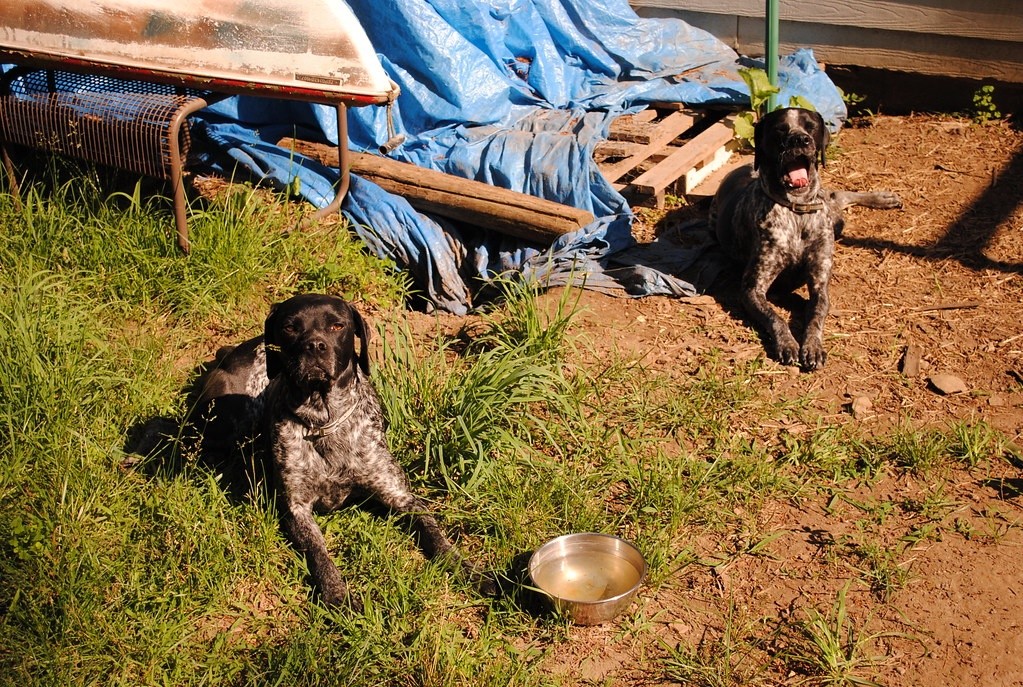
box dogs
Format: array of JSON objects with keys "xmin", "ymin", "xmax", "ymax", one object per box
[
  {"xmin": 190, "ymin": 293, "xmax": 475, "ymax": 617},
  {"xmin": 711, "ymin": 107, "xmax": 903, "ymax": 369}
]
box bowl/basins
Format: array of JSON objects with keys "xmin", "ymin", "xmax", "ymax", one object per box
[{"xmin": 527, "ymin": 532, "xmax": 647, "ymax": 626}]
